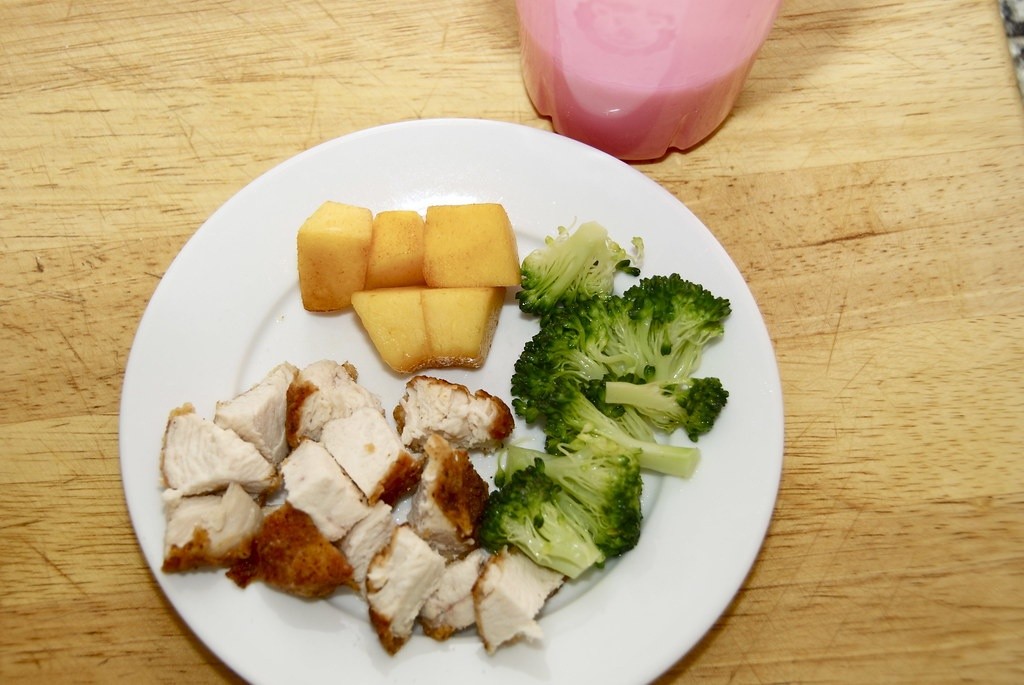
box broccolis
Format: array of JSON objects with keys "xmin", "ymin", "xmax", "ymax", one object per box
[{"xmin": 478, "ymin": 221, "xmax": 732, "ymax": 580}]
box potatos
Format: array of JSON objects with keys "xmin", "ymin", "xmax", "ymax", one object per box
[{"xmin": 297, "ymin": 200, "xmax": 520, "ymax": 374}]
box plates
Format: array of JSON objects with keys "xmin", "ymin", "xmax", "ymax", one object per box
[{"xmin": 118, "ymin": 118, "xmax": 784, "ymax": 685}]
